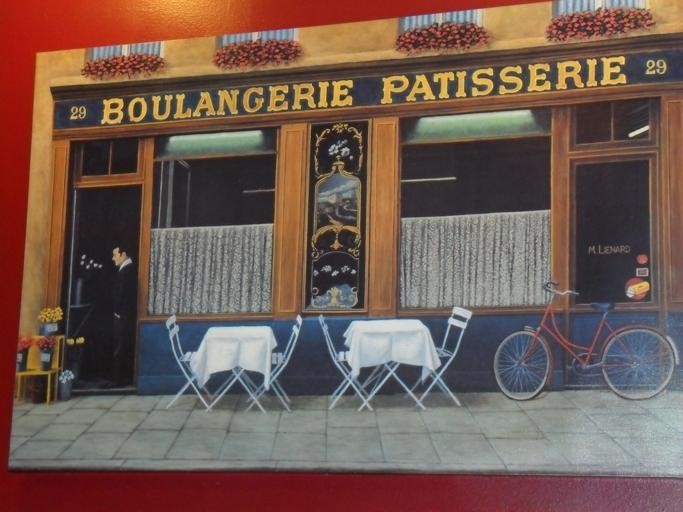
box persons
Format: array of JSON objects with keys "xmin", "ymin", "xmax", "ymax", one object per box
[{"xmin": 104, "ymin": 246, "xmax": 136, "ymax": 387}]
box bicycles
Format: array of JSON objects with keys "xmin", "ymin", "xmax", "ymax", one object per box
[{"xmin": 493, "ymin": 281, "xmax": 681, "ymax": 401}]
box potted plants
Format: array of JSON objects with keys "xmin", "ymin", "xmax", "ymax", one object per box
[
  {"xmin": 15, "ymin": 335, "xmax": 33, "ymax": 371},
  {"xmin": 36, "ymin": 307, "xmax": 63, "ymax": 334},
  {"xmin": 36, "ymin": 335, "xmax": 57, "ymax": 371},
  {"xmin": 58, "ymin": 369, "xmax": 75, "ymax": 401}
]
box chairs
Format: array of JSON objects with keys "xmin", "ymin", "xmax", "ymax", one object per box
[
  {"xmin": 14, "ymin": 335, "xmax": 65, "ymax": 406},
  {"xmin": 317, "ymin": 313, "xmax": 375, "ymax": 412},
  {"xmin": 165, "ymin": 314, "xmax": 214, "ymax": 413},
  {"xmin": 404, "ymin": 306, "xmax": 474, "ymax": 410},
  {"xmin": 245, "ymin": 314, "xmax": 303, "ymax": 413}
]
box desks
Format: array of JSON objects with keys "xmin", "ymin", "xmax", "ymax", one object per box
[
  {"xmin": 343, "ymin": 319, "xmax": 442, "ymax": 413},
  {"xmin": 189, "ymin": 324, "xmax": 278, "ymax": 415}
]
